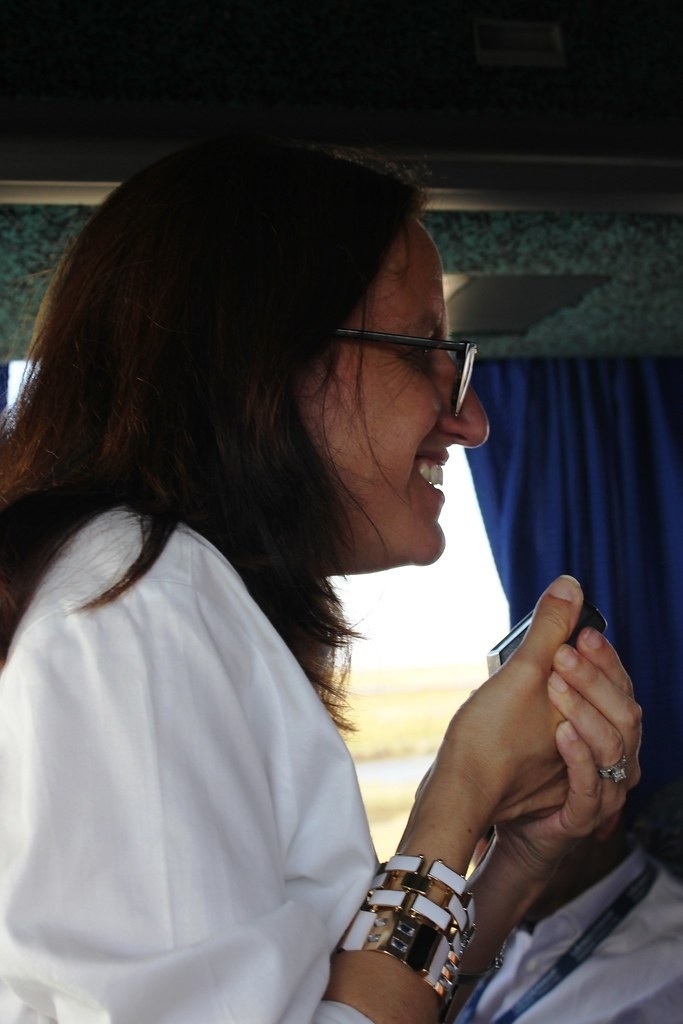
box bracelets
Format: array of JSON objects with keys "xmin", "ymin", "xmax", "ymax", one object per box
[{"xmin": 337, "ymin": 853, "xmax": 477, "ymax": 1007}]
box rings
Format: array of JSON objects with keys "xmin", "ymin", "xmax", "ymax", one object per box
[{"xmin": 599, "ymin": 755, "xmax": 627, "ymax": 783}]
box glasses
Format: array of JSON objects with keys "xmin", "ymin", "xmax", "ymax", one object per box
[{"xmin": 335, "ymin": 328, "xmax": 478, "ymax": 417}]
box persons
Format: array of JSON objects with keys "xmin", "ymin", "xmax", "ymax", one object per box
[
  {"xmin": 0, "ymin": 138, "xmax": 641, "ymax": 1024},
  {"xmin": 454, "ymin": 788, "xmax": 683, "ymax": 1024}
]
